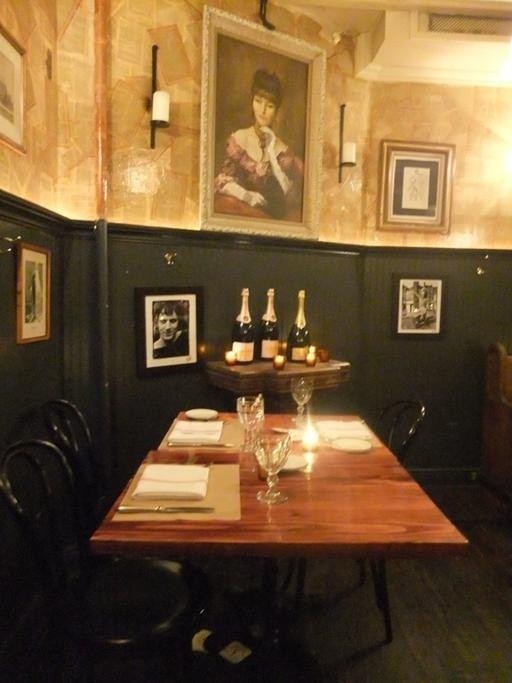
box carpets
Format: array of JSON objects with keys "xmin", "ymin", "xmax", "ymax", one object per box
[{"xmin": 419, "ymin": 483, "xmax": 508, "ymax": 523}]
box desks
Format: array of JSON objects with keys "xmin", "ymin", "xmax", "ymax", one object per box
[
  {"xmin": 85, "ymin": 405, "xmax": 470, "ymax": 682},
  {"xmin": 202, "ymin": 355, "xmax": 355, "ymax": 417}
]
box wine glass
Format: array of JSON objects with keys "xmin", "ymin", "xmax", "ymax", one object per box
[
  {"xmin": 290, "ymin": 375, "xmax": 313, "ymax": 439},
  {"xmin": 236, "ymin": 394, "xmax": 290, "ymax": 506}
]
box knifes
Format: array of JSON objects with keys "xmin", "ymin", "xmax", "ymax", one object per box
[
  {"xmin": 161, "ymin": 441, "xmax": 233, "ymax": 449},
  {"xmin": 117, "ymin": 501, "xmax": 214, "ymax": 513}
]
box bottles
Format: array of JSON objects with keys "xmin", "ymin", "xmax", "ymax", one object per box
[
  {"xmin": 229, "ymin": 286, "xmax": 255, "ymax": 364},
  {"xmin": 259, "ymin": 288, "xmax": 282, "ymax": 361},
  {"xmin": 287, "ymin": 289, "xmax": 313, "ymax": 362}
]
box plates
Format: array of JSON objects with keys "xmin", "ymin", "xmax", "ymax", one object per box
[
  {"xmin": 277, "ymin": 453, "xmax": 309, "ymax": 472},
  {"xmin": 187, "ymin": 406, "xmax": 218, "ymax": 421},
  {"xmin": 331, "ymin": 436, "xmax": 373, "ymax": 456}
]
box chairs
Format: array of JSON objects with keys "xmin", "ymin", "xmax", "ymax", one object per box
[
  {"xmin": 0, "ymin": 437, "xmax": 217, "ymax": 682},
  {"xmin": 40, "ymin": 398, "xmax": 116, "ymax": 549},
  {"xmin": 282, "ymin": 397, "xmax": 431, "ymax": 642}
]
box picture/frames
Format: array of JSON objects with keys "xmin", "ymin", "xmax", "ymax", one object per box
[
  {"xmin": 375, "ymin": 138, "xmax": 457, "ymax": 237},
  {"xmin": 195, "ymin": 3, "xmax": 326, "ymax": 244},
  {"xmin": 387, "ymin": 268, "xmax": 449, "ymax": 341},
  {"xmin": 13, "ymin": 238, "xmax": 53, "ymax": 345},
  {"xmin": 133, "ymin": 285, "xmax": 203, "ymax": 375},
  {"xmin": 1, "ymin": 20, "xmax": 30, "ymax": 158}
]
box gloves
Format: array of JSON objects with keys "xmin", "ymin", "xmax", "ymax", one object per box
[
  {"xmin": 220, "ymin": 180, "xmax": 269, "ymax": 210},
  {"xmin": 261, "ymin": 125, "xmax": 293, "ymax": 191}
]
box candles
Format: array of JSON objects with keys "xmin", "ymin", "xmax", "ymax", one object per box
[
  {"xmin": 342, "ymin": 141, "xmax": 356, "ymax": 163},
  {"xmin": 151, "ymin": 90, "xmax": 171, "ymax": 120}
]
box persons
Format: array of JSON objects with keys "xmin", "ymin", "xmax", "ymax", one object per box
[
  {"xmin": 213, "ymin": 67, "xmax": 303, "ymax": 221},
  {"xmin": 152, "ymin": 302, "xmax": 188, "ymax": 359}
]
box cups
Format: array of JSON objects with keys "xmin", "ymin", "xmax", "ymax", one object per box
[
  {"xmin": 224, "ymin": 351, "xmax": 237, "ymax": 365},
  {"xmin": 304, "ymin": 357, "xmax": 318, "ymax": 369},
  {"xmin": 319, "ymin": 348, "xmax": 332, "ymax": 363},
  {"xmin": 272, "ymin": 357, "xmax": 287, "ymax": 371}
]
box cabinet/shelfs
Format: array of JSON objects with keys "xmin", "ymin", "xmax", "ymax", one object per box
[{"xmin": 480, "ymin": 341, "xmax": 511, "ymax": 498}]
